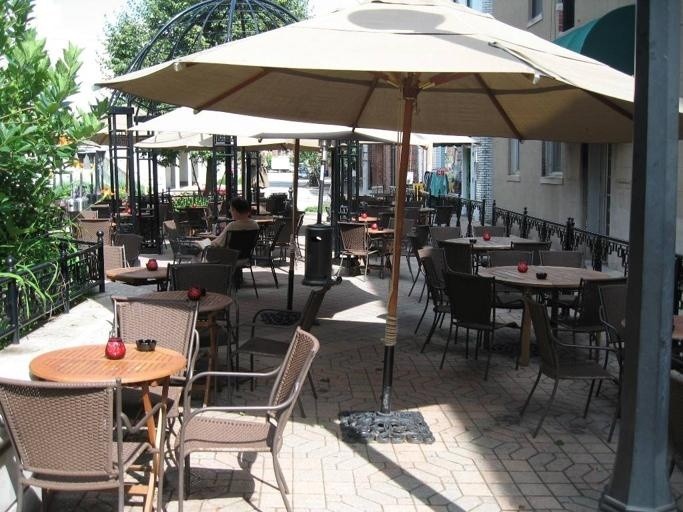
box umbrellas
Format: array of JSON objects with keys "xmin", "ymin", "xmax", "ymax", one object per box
[{"xmin": 92, "ymin": 1, "xmax": 682, "ymax": 427}]
